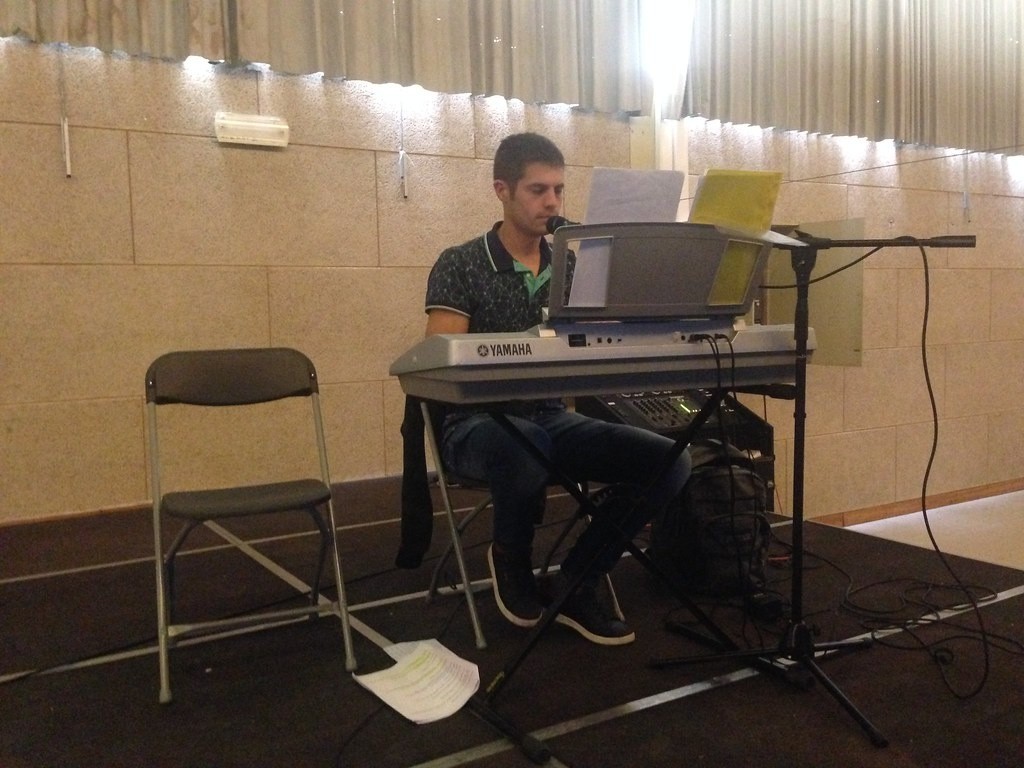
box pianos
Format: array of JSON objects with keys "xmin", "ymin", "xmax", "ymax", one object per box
[{"xmin": 387, "ymin": 323, "xmax": 820, "ymax": 404}]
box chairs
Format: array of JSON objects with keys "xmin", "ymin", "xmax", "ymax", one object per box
[
  {"xmin": 420, "ymin": 399, "xmax": 627, "ymax": 650},
  {"xmin": 146, "ymin": 347, "xmax": 357, "ymax": 707}
]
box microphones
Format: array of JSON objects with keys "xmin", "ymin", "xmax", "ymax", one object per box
[{"xmin": 546, "ymin": 216, "xmax": 581, "ymax": 234}]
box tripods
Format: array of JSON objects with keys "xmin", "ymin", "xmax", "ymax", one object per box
[{"xmin": 647, "ymin": 234, "xmax": 977, "ymax": 748}]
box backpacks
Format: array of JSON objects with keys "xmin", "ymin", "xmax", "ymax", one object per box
[{"xmin": 641, "ymin": 439, "xmax": 772, "ymax": 598}]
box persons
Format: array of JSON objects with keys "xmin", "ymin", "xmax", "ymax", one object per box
[{"xmin": 424, "ymin": 133, "xmax": 693, "ymax": 640}]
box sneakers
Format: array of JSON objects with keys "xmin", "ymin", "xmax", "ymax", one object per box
[
  {"xmin": 487, "ymin": 542, "xmax": 543, "ymax": 628},
  {"xmin": 556, "ymin": 590, "xmax": 636, "ymax": 646}
]
